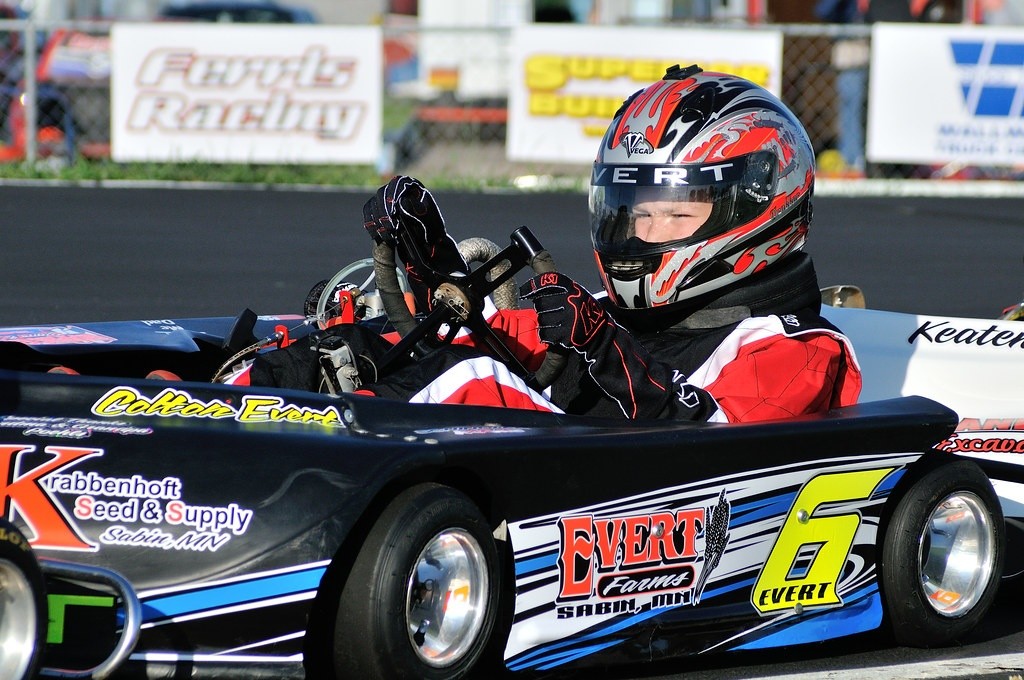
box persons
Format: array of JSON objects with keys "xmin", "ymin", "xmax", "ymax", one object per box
[{"xmin": 46, "ymin": 65, "xmax": 862, "ymax": 425}]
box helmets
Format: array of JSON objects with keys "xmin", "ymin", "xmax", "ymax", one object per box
[{"xmin": 588, "ymin": 63, "xmax": 816, "ymax": 329}]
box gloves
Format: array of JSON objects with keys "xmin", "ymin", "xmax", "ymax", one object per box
[
  {"xmin": 520, "ymin": 273, "xmax": 611, "ymax": 351},
  {"xmin": 363, "ymin": 176, "xmax": 446, "ymax": 250}
]
